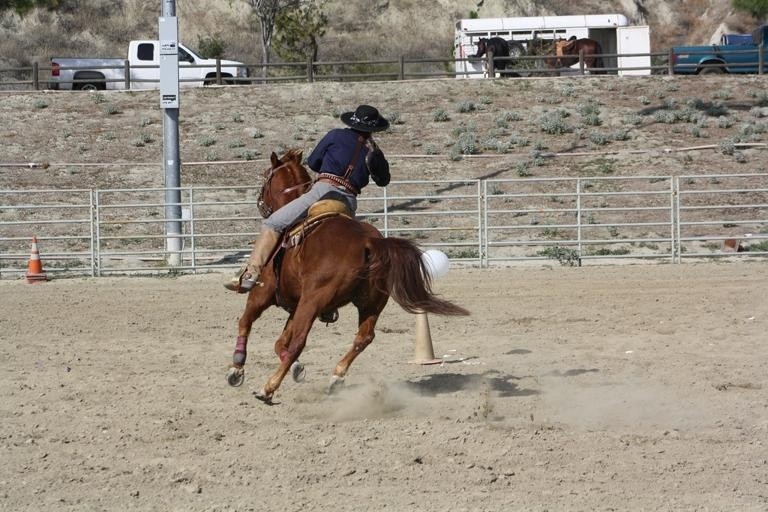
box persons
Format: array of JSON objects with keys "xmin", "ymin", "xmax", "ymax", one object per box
[{"xmin": 220, "ymin": 104, "xmax": 391, "ymax": 291}]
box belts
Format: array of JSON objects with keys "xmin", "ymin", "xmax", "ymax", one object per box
[{"xmin": 318, "ymin": 177, "xmax": 354, "ymax": 193}]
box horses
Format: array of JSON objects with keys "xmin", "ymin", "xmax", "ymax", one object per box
[
  {"xmin": 475, "ymin": 36, "xmax": 527, "ymax": 78},
  {"xmin": 525, "ymin": 39, "xmax": 607, "ymax": 77},
  {"xmin": 226, "ymin": 146, "xmax": 471, "ymax": 404}
]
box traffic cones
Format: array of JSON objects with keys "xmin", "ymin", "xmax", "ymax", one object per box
[
  {"xmin": 25, "ymin": 235, "xmax": 46, "ymax": 281},
  {"xmin": 406, "ymin": 307, "xmax": 442, "ymax": 365}
]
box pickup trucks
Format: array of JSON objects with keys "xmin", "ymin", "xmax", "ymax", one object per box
[
  {"xmin": 47, "ymin": 40, "xmax": 251, "ymax": 90},
  {"xmin": 667, "ymin": 25, "xmax": 767, "ymax": 74}
]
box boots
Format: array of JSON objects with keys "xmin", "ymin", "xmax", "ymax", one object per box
[
  {"xmin": 322, "ymin": 313, "xmax": 333, "ymax": 320},
  {"xmin": 223, "ymin": 227, "xmax": 281, "ymax": 294}
]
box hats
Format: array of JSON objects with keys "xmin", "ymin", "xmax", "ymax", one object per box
[{"xmin": 340, "ymin": 104, "xmax": 390, "ymax": 132}]
